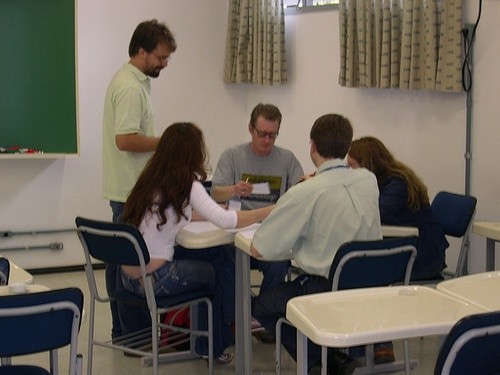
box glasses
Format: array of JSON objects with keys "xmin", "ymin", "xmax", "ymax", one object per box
[
  {"xmin": 150, "ymin": 52, "xmax": 168, "ymax": 61},
  {"xmin": 252, "ymin": 125, "xmax": 278, "ymax": 139}
]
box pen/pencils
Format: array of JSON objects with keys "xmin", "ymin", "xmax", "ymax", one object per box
[
  {"xmin": 296, "ymin": 172, "xmax": 316, "ymax": 184},
  {"xmin": 241, "ymin": 177, "xmax": 249, "ymax": 194}
]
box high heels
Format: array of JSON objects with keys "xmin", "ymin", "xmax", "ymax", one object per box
[
  {"xmin": 250, "ymin": 317, "xmax": 266, "ymax": 341},
  {"xmin": 202, "ymin": 352, "xmax": 233, "ymax": 369}
]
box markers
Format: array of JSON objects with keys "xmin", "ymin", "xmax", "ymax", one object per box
[{"xmin": 0, "ymin": 146, "xmax": 43, "ymax": 155}]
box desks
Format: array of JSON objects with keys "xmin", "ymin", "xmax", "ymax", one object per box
[{"xmin": 178, "ymin": 216, "xmax": 500, "ymax": 375}]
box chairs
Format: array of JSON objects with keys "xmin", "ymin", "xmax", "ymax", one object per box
[
  {"xmin": 276, "ymin": 234, "xmax": 419, "ymax": 375},
  {"xmin": 0, "ymin": 287, "xmax": 84, "ymax": 375},
  {"xmin": 395, "ymin": 190, "xmax": 479, "ymax": 286},
  {"xmin": 435, "ymin": 311, "xmax": 500, "ymax": 375},
  {"xmin": 75, "ymin": 215, "xmax": 213, "ymax": 375}
]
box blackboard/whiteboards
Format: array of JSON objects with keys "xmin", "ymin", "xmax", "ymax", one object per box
[{"xmin": 0, "ymin": 0, "xmax": 80, "ymax": 159}]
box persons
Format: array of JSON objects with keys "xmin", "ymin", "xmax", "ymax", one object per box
[
  {"xmin": 210, "ymin": 103, "xmax": 304, "ymax": 343},
  {"xmin": 250, "ymin": 112, "xmax": 383, "ymax": 375},
  {"xmin": 116, "ymin": 122, "xmax": 275, "ymax": 366},
  {"xmin": 345, "ymin": 137, "xmax": 450, "ymax": 366},
  {"xmin": 102, "ymin": 18, "xmax": 178, "ymax": 357}
]
